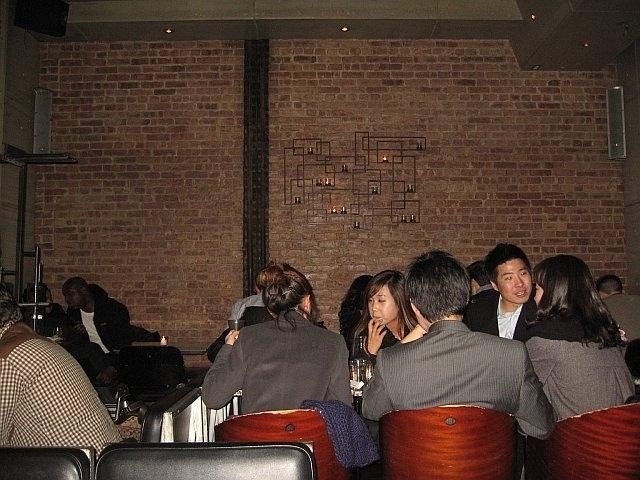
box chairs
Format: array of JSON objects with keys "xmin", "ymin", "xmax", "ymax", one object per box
[
  {"xmin": 1, "ymin": 334, "xmax": 359, "ymax": 479},
  {"xmin": 542, "ymin": 401, "xmax": 639, "ymax": 480},
  {"xmin": 375, "ymin": 403, "xmax": 518, "ymax": 479}
]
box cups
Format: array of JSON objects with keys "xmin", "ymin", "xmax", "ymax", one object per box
[
  {"xmin": 227, "ymin": 319, "xmax": 246, "ymax": 333},
  {"xmin": 348, "ymin": 359, "xmax": 374, "ymax": 383},
  {"xmin": 53, "ymin": 326, "xmax": 63, "ymax": 340}
]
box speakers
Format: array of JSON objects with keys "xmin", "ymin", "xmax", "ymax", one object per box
[
  {"xmin": 33, "ymin": 88, "xmax": 53, "ymax": 155},
  {"xmin": 605, "ymin": 85, "xmax": 626, "ymax": 159},
  {"xmin": 14, "ymin": 0, "xmax": 69, "ymax": 37}
]
